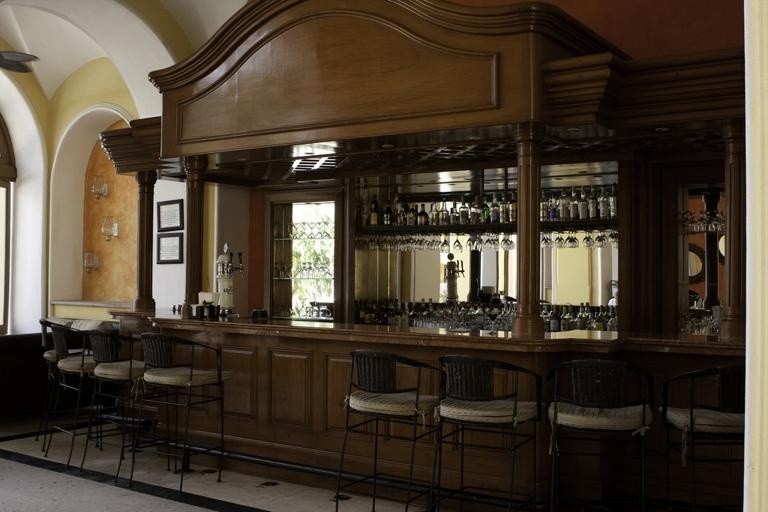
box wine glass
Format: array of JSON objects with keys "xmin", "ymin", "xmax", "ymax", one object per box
[
  {"xmin": 682, "ymin": 208, "xmax": 730, "ymax": 235},
  {"xmin": 286, "ymin": 222, "xmax": 333, "ymax": 239},
  {"xmin": 676, "ymin": 307, "xmax": 719, "ymax": 338},
  {"xmin": 539, "ymin": 224, "xmax": 618, "ymax": 252},
  {"xmin": 350, "ymin": 227, "xmax": 517, "ymax": 255},
  {"xmin": 273, "ymin": 257, "xmax": 334, "ymax": 279},
  {"xmin": 277, "ymin": 301, "xmax": 331, "ymax": 319}
]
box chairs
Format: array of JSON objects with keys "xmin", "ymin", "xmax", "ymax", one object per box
[{"xmin": 32, "ymin": 312, "xmax": 232, "ymax": 499}]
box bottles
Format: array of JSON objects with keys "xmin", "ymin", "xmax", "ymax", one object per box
[
  {"xmin": 353, "ymin": 177, "xmax": 518, "ymax": 226},
  {"xmin": 539, "ymin": 299, "xmax": 619, "ymax": 333},
  {"xmin": 539, "ymin": 182, "xmax": 617, "ymax": 220},
  {"xmin": 354, "ymin": 294, "xmax": 517, "ymax": 332}
]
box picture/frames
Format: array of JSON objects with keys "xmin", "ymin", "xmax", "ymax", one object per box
[
  {"xmin": 156, "ymin": 199, "xmax": 185, "ymax": 231},
  {"xmin": 155, "ymin": 233, "xmax": 184, "ymax": 266}
]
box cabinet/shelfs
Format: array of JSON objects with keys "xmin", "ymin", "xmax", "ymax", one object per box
[{"xmin": 272, "ymin": 234, "xmax": 334, "ymax": 322}]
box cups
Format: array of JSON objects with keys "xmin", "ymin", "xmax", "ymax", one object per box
[{"xmin": 182, "ymin": 304, "xmax": 193, "ymax": 319}]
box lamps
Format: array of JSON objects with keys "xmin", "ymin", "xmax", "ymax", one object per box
[
  {"xmin": 100, "ymin": 216, "xmax": 119, "ymax": 242},
  {"xmin": 90, "ymin": 174, "xmax": 106, "ymax": 200},
  {"xmin": 83, "ymin": 250, "xmax": 101, "ymax": 274}
]
